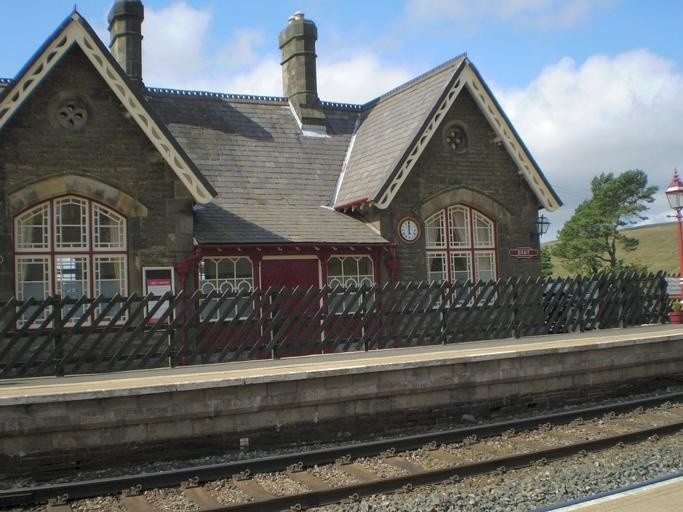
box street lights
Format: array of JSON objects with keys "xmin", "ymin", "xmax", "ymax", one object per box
[{"xmin": 665, "ymin": 168, "xmax": 683, "ymax": 324}]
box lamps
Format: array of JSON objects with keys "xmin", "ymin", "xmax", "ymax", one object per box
[{"xmin": 530, "ymin": 214, "xmax": 551, "ymax": 238}]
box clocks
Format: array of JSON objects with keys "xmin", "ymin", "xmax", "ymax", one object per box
[{"xmin": 397, "ymin": 216, "xmax": 422, "ymax": 244}]
